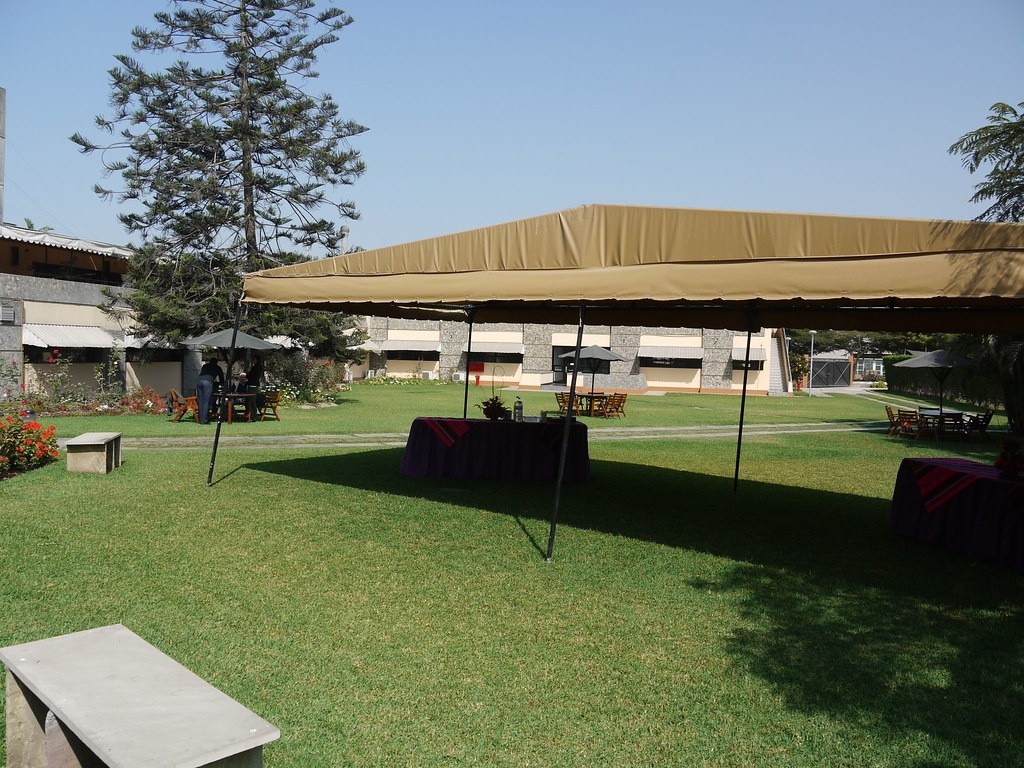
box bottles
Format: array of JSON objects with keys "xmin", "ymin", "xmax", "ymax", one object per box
[{"xmin": 514, "ymin": 395, "xmax": 523, "ymax": 422}]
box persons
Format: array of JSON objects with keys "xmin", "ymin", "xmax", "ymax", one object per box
[
  {"xmin": 246, "ymin": 353, "xmax": 262, "ymax": 422},
  {"xmin": 198, "ymin": 357, "xmax": 224, "ymax": 424},
  {"xmin": 226, "ymin": 373, "xmax": 247, "ymax": 413}
]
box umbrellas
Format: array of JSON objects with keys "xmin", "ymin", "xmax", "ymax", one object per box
[
  {"xmin": 891, "ymin": 350, "xmax": 987, "ymax": 412},
  {"xmin": 179, "ymin": 328, "xmax": 280, "ymax": 390},
  {"xmin": 559, "ymin": 344, "xmax": 627, "ymax": 395}
]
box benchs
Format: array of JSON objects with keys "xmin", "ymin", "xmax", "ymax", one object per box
[
  {"xmin": 65, "ymin": 432, "xmax": 123, "ymax": 474},
  {"xmin": 0, "ymin": 625, "xmax": 281, "ymax": 768}
]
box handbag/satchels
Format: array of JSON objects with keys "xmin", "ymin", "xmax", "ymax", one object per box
[{"xmin": 256, "ymin": 392, "xmax": 266, "ymax": 407}]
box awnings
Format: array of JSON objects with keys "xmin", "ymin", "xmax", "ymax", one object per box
[
  {"xmin": 462, "ymin": 342, "xmax": 524, "ymax": 354},
  {"xmin": 381, "ymin": 340, "xmax": 440, "ymax": 351},
  {"xmin": 638, "ymin": 346, "xmax": 703, "ymax": 359},
  {"xmin": 732, "ymin": 348, "xmax": 767, "ymax": 360},
  {"xmin": 23, "ymin": 324, "xmax": 124, "ymax": 348}
]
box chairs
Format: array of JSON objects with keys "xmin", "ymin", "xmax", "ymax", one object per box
[
  {"xmin": 885, "ymin": 405, "xmax": 994, "ymax": 445},
  {"xmin": 555, "ymin": 392, "xmax": 627, "ymax": 420},
  {"xmin": 169, "ymin": 386, "xmax": 280, "ymax": 422}
]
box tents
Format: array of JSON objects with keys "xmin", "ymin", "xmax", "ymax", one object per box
[{"xmin": 206, "ymin": 204, "xmax": 1024, "ymax": 564}]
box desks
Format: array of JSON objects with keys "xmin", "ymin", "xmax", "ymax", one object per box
[
  {"xmin": 400, "ymin": 417, "xmax": 590, "ymax": 480},
  {"xmin": 889, "ymin": 457, "xmax": 1024, "ymax": 572},
  {"xmin": 918, "ymin": 410, "xmax": 963, "ymax": 434},
  {"xmin": 576, "ymin": 394, "xmax": 613, "ymax": 415},
  {"xmin": 211, "ymin": 392, "xmax": 257, "ymax": 419}
]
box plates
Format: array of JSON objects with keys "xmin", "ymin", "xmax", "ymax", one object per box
[{"xmin": 523, "ymin": 416, "xmax": 542, "ymax": 422}]
